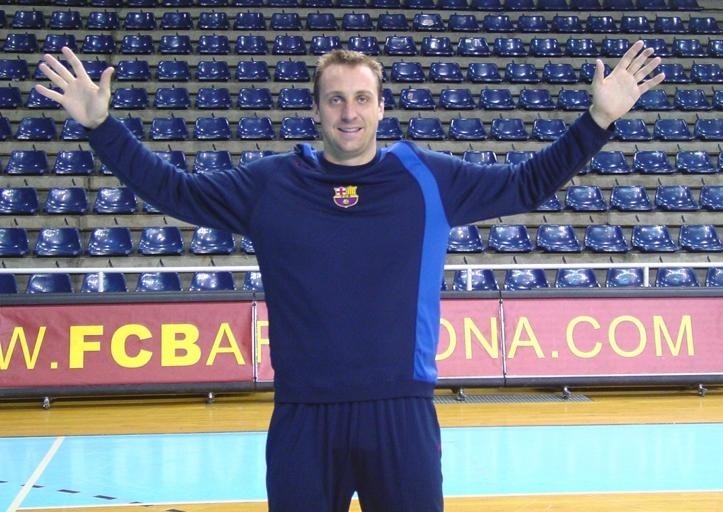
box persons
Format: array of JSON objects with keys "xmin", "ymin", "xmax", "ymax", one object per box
[{"xmin": 32, "ymin": 40, "xmax": 666, "ymax": 512}]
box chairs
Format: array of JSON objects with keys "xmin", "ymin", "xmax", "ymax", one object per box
[{"xmin": 0, "ymin": 1, "xmax": 722, "ymax": 298}]
